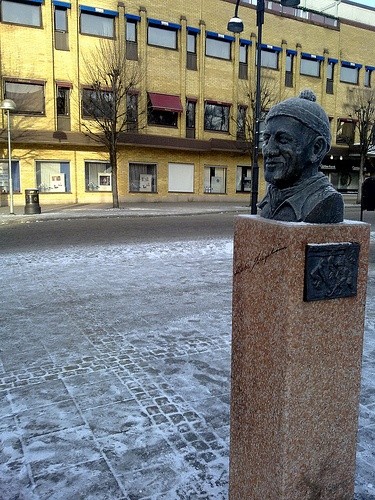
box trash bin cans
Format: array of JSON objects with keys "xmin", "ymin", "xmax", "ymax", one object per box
[{"xmin": 23, "ymin": 188, "xmax": 41, "ymax": 214}]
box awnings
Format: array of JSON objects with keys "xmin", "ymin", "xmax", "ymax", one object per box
[{"xmin": 147, "ymin": 91, "xmax": 183, "ymax": 113}]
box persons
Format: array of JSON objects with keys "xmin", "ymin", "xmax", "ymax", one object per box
[{"xmin": 256, "ymin": 90, "xmax": 344, "ymax": 225}]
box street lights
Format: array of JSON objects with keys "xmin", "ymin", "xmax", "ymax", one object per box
[
  {"xmin": 0, "ymin": 98, "xmax": 17, "ymax": 216},
  {"xmin": 226, "ymin": 0, "xmax": 300, "ymax": 217}
]
